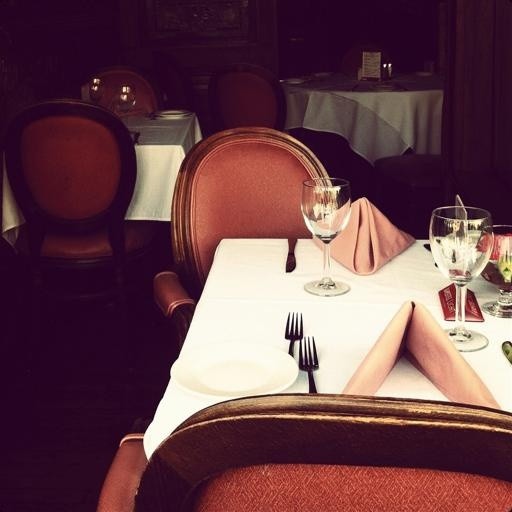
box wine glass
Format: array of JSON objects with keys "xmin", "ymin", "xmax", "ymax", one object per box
[
  {"xmin": 479, "ymin": 223, "xmax": 512, "ymax": 319},
  {"xmin": 299, "ymin": 176, "xmax": 353, "ymax": 297},
  {"xmin": 87, "ymin": 74, "xmax": 104, "ymax": 107},
  {"xmin": 429, "ymin": 205, "xmax": 496, "ymax": 354},
  {"xmin": 117, "ymin": 82, "xmax": 137, "ymax": 124}
]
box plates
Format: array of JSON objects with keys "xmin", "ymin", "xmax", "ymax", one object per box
[
  {"xmin": 284, "ymin": 79, "xmax": 304, "ymax": 85},
  {"xmin": 168, "ymin": 340, "xmax": 299, "ymax": 400},
  {"xmin": 160, "ymin": 109, "xmax": 191, "ymax": 118}
]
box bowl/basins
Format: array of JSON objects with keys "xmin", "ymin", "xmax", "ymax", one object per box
[{"xmin": 475, "ymin": 222, "xmax": 512, "ymax": 284}]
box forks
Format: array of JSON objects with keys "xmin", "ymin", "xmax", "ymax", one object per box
[
  {"xmin": 297, "ymin": 335, "xmax": 321, "ymax": 394},
  {"xmin": 282, "ymin": 312, "xmax": 304, "ymax": 357}
]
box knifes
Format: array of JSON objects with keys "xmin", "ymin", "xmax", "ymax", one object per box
[{"xmin": 284, "ymin": 236, "xmax": 299, "ymax": 273}]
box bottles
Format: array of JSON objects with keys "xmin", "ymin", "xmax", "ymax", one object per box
[{"xmin": 381, "ymin": 60, "xmax": 394, "ymax": 80}]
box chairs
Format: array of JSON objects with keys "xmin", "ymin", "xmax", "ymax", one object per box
[
  {"xmin": 155, "ymin": 128, "xmax": 332, "ymax": 341},
  {"xmin": 132, "ymin": 399, "xmax": 511, "ymax": 511},
  {"xmin": 199, "ymin": 68, "xmax": 290, "ymax": 129},
  {"xmin": 3, "ymin": 101, "xmax": 135, "ymax": 307},
  {"xmin": 81, "ymin": 70, "xmax": 154, "ymax": 109}
]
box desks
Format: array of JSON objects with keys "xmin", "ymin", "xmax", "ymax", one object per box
[
  {"xmin": 137, "ymin": 236, "xmax": 510, "ymax": 461},
  {"xmin": 282, "ymin": 65, "xmax": 451, "ymax": 156},
  {"xmin": 0, "ymin": 107, "xmax": 197, "ymax": 230}
]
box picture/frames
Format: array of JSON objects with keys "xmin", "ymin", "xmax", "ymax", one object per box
[{"xmin": 143, "ymin": 0, "xmax": 278, "ymax": 49}]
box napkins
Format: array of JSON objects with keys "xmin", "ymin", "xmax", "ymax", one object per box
[
  {"xmin": 338, "ymin": 298, "xmax": 495, "ymax": 408},
  {"xmin": 311, "ymin": 194, "xmax": 415, "ymax": 274}
]
